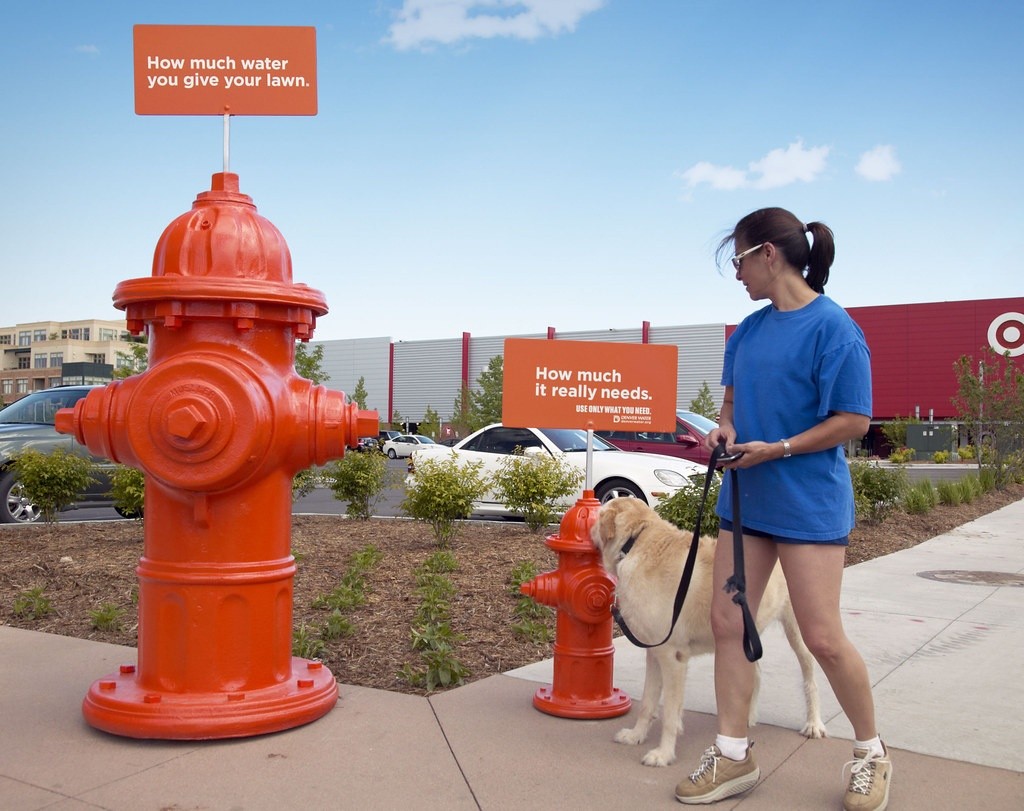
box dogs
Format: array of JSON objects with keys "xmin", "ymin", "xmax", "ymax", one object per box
[{"xmin": 589, "ymin": 496, "xmax": 826, "ymax": 766}]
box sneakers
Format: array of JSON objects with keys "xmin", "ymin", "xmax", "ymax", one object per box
[
  {"xmin": 674, "ymin": 739, "xmax": 760, "ymax": 804},
  {"xmin": 841, "ymin": 733, "xmax": 892, "ymax": 811}
]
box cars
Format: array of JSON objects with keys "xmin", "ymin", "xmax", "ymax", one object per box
[
  {"xmin": 404, "ymin": 415, "xmax": 724, "ymax": 525},
  {"xmin": 0, "ymin": 383, "xmax": 144, "ymax": 524},
  {"xmin": 346, "ymin": 436, "xmax": 380, "ymax": 453},
  {"xmin": 597, "ymin": 407, "xmax": 730, "ymax": 473},
  {"xmin": 382, "ymin": 434, "xmax": 446, "ymax": 460},
  {"xmin": 439, "ymin": 438, "xmax": 475, "ymax": 450}
]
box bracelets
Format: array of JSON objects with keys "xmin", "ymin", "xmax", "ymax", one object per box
[{"xmin": 780, "ymin": 439, "xmax": 791, "ymax": 458}]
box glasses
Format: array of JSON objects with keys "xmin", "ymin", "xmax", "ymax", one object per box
[{"xmin": 732, "ymin": 243, "xmax": 763, "ymax": 270}]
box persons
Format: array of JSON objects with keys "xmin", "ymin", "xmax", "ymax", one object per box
[{"xmin": 674, "ymin": 207, "xmax": 893, "ymax": 811}]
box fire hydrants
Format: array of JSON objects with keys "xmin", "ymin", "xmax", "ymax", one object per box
[
  {"xmin": 54, "ymin": 171, "xmax": 380, "ymax": 741},
  {"xmin": 520, "ymin": 489, "xmax": 632, "ymax": 720}
]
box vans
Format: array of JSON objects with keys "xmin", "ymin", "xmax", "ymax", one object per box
[{"xmin": 378, "ymin": 428, "xmax": 405, "ymax": 451}]
